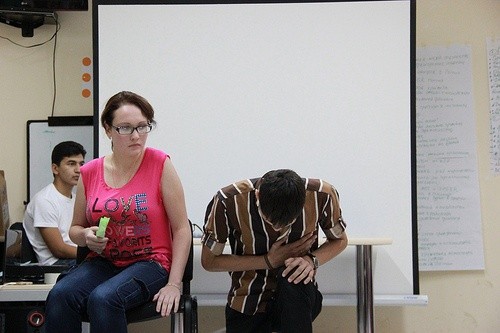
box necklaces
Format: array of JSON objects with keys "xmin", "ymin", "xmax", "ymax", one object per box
[{"xmin": 110, "ymin": 150, "xmax": 143, "ymax": 189}]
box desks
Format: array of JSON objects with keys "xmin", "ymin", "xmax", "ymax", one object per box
[{"xmin": 193, "ymin": 237, "xmax": 394, "ymax": 333}]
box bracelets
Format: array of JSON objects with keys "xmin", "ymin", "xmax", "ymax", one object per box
[
  {"xmin": 263, "ymin": 252, "xmax": 276, "ymax": 271},
  {"xmin": 166, "ymin": 281, "xmax": 183, "ymax": 295}
]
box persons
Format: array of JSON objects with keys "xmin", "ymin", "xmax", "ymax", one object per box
[
  {"xmin": 22, "ymin": 140, "xmax": 88, "ymax": 278},
  {"xmin": 200, "ymin": 167, "xmax": 348, "ymax": 333},
  {"xmin": 43, "ymin": 90, "xmax": 192, "ymax": 333}
]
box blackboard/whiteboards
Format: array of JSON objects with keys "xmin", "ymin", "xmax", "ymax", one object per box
[{"xmin": 23, "ymin": 116, "xmax": 94, "ymax": 205}]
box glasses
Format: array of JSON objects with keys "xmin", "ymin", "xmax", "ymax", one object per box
[{"xmin": 112, "ymin": 123, "xmax": 152, "ymax": 135}]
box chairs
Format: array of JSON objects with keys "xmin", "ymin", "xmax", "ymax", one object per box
[{"xmin": 0, "ymin": 218, "xmax": 198, "ymax": 333}]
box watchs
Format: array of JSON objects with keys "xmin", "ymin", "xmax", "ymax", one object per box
[{"xmin": 304, "ymin": 252, "xmax": 320, "ymax": 270}]
